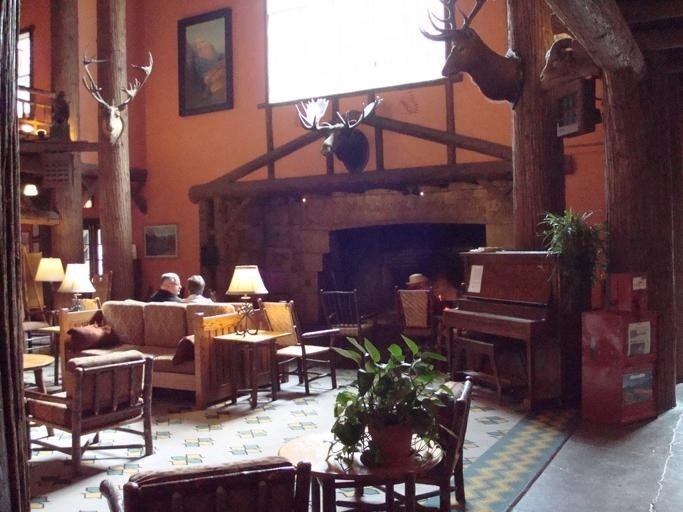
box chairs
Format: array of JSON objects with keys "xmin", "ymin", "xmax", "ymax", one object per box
[
  {"xmin": 392, "ymin": 283, "xmax": 445, "ymax": 366},
  {"xmin": 98, "ymin": 457, "xmax": 313, "ymax": 512},
  {"xmin": 22, "ymin": 348, "xmax": 154, "ymax": 473},
  {"xmin": 319, "ymin": 287, "xmax": 387, "ymax": 376},
  {"xmin": 255, "ymin": 297, "xmax": 340, "ymax": 396},
  {"xmin": 388, "ymin": 374, "xmax": 474, "ymax": 511}
]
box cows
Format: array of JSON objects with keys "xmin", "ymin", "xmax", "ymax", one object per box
[{"xmin": 538, "ymin": 34, "xmax": 603, "ymax": 86}]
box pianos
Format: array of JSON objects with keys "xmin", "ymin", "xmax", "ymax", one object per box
[{"xmin": 442, "ymin": 247, "xmax": 565, "ymax": 417}]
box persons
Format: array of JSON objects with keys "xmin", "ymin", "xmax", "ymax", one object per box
[
  {"xmin": 182, "ymin": 275, "xmax": 213, "ymax": 302},
  {"xmin": 402, "ymin": 274, "xmax": 446, "ymax": 328},
  {"xmin": 149, "ymin": 272, "xmax": 182, "ymax": 302}
]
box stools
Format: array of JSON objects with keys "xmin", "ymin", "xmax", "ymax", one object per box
[{"xmin": 455, "ymin": 335, "xmax": 519, "ymax": 404}]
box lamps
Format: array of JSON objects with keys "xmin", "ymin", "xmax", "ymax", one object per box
[
  {"xmin": 55, "ymin": 263, "xmax": 97, "ymax": 310},
  {"xmin": 226, "ymin": 264, "xmax": 267, "ymax": 336},
  {"xmin": 32, "ymin": 256, "xmax": 64, "ymax": 283}
]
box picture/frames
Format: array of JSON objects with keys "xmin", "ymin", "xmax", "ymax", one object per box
[
  {"xmin": 176, "ymin": 8, "xmax": 232, "ymax": 117},
  {"xmin": 142, "ymin": 223, "xmax": 178, "ymax": 259}
]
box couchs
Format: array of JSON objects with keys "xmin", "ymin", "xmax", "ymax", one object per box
[{"xmin": 59, "ymin": 299, "xmax": 247, "ymax": 411}]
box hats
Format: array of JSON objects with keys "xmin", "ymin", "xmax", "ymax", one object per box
[{"xmin": 405, "ymin": 274, "xmax": 428, "ymax": 285}]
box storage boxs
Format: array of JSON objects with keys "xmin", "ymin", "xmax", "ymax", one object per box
[{"xmin": 581, "ymin": 272, "xmax": 657, "ymax": 425}]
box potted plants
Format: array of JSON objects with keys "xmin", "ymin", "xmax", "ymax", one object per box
[
  {"xmin": 535, "ymin": 208, "xmax": 612, "ymax": 285},
  {"xmin": 316, "ymin": 331, "xmax": 458, "ymax": 470}
]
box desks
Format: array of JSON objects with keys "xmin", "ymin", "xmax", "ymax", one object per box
[
  {"xmin": 27, "ymin": 325, "xmax": 61, "ymax": 386},
  {"xmin": 278, "ymin": 428, "xmax": 445, "ymax": 512},
  {"xmin": 23, "ymin": 353, "xmax": 56, "ymax": 437},
  {"xmin": 211, "ymin": 329, "xmax": 292, "ymax": 410}
]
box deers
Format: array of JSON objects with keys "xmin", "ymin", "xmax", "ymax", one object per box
[
  {"xmin": 80, "ymin": 53, "xmax": 154, "ymax": 149},
  {"xmin": 293, "ymin": 93, "xmax": 386, "ymax": 172},
  {"xmin": 420, "ymin": 1, "xmax": 525, "ymax": 107}
]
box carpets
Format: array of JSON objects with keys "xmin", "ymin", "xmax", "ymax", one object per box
[{"xmin": 14, "ymin": 347, "xmax": 579, "ymax": 512}]
